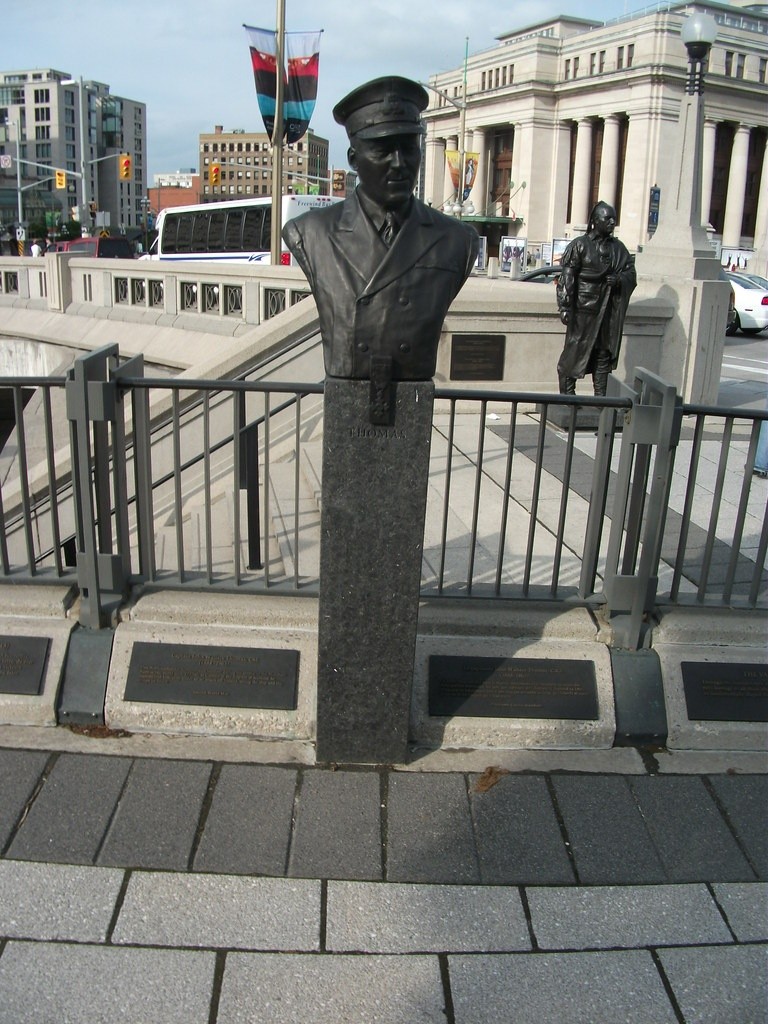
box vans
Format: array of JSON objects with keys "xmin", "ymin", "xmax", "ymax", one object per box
[{"xmin": 67, "ymin": 237, "xmax": 134, "ymax": 259}]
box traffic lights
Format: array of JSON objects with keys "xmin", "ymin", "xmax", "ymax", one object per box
[
  {"xmin": 208, "ymin": 163, "xmax": 222, "ymax": 186},
  {"xmin": 72, "ymin": 205, "xmax": 81, "ymax": 224},
  {"xmin": 55, "ymin": 170, "xmax": 66, "ymax": 189},
  {"xmin": 118, "ymin": 154, "xmax": 132, "ymax": 181}
]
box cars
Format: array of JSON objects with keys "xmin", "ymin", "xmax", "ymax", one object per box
[
  {"xmin": 726, "ymin": 271, "xmax": 768, "ymax": 334},
  {"xmin": 140, "ymin": 237, "xmax": 158, "ymax": 261},
  {"xmin": 515, "ymin": 253, "xmax": 736, "ymax": 335},
  {"xmin": 42, "ymin": 241, "xmax": 70, "ymax": 256}
]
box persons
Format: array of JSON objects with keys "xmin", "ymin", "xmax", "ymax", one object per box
[
  {"xmin": 503, "ymin": 246, "xmax": 523, "ymax": 264},
  {"xmin": 31, "ymin": 237, "xmax": 55, "ymax": 258},
  {"xmin": 526, "ymin": 249, "xmax": 540, "ymax": 266},
  {"xmin": 283, "ymin": 75, "xmax": 480, "ymax": 383},
  {"xmin": 556, "ymin": 200, "xmax": 636, "ymax": 411}
]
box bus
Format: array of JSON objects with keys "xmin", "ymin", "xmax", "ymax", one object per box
[{"xmin": 156, "ymin": 194, "xmax": 347, "ymax": 268}]
